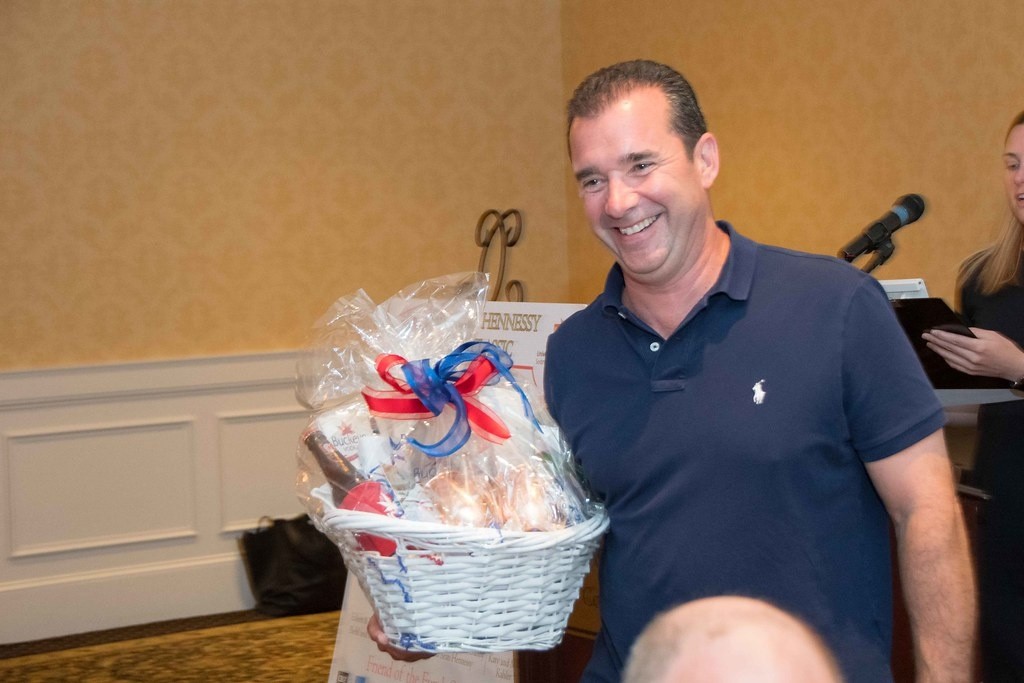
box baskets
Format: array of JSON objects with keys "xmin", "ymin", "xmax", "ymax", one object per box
[{"xmin": 310, "ymin": 481, "xmax": 611, "ymax": 652}]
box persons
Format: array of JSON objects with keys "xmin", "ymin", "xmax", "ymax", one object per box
[
  {"xmin": 542, "ymin": 59, "xmax": 979, "ymax": 683},
  {"xmin": 921, "ymin": 110, "xmax": 1023, "ymax": 504}
]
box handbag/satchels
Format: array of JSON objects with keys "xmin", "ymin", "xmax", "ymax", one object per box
[{"xmin": 238, "ymin": 513, "xmax": 348, "ymax": 618}]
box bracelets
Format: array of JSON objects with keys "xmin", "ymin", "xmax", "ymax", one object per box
[{"xmin": 1009, "ymin": 379, "xmax": 1023, "ymax": 390}]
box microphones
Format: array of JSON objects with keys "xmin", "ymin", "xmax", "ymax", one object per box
[{"xmin": 840, "ymin": 194, "xmax": 925, "ymax": 262}]
